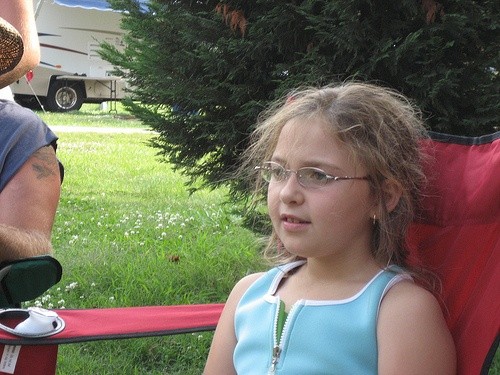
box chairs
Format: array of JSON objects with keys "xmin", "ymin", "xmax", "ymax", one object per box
[{"xmin": 0, "ymin": 132, "xmax": 500, "ymax": 375}]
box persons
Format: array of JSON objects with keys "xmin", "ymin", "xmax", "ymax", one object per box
[
  {"xmin": 201, "ymin": 80, "xmax": 457, "ymax": 375},
  {"xmin": 0, "ymin": 0, "xmax": 64, "ymax": 263}
]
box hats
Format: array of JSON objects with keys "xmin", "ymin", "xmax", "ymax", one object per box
[{"xmin": 1, "ymin": 17, "xmax": 24, "ymax": 77}]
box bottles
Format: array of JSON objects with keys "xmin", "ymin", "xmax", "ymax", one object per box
[{"xmin": 13, "ymin": 306, "xmax": 58, "ymax": 336}]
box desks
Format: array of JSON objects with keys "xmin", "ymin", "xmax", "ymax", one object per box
[{"xmin": 56, "ymin": 76, "xmax": 122, "ymax": 114}]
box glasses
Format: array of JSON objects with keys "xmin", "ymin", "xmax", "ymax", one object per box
[{"xmin": 254, "ymin": 161, "xmax": 370, "ymax": 191}]
